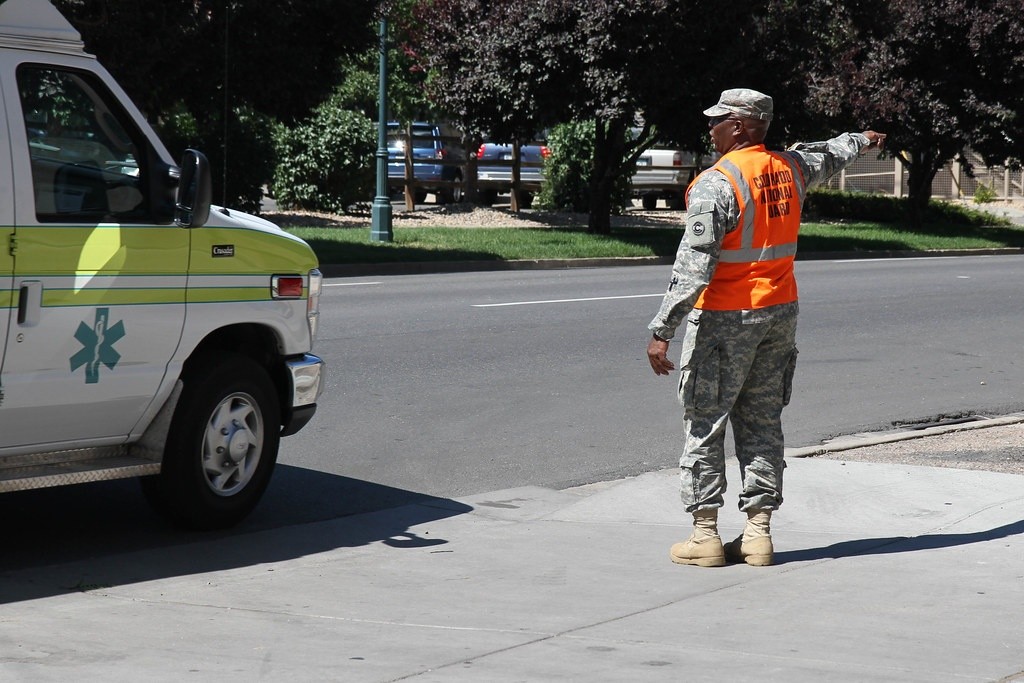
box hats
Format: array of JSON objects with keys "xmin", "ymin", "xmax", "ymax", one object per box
[{"xmin": 703, "ymin": 88, "xmax": 773, "ymax": 122}]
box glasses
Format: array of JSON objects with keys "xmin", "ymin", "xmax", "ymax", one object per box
[{"xmin": 710, "ymin": 116, "xmax": 742, "ymax": 125}]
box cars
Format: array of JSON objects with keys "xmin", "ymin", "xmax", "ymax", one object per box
[
  {"xmin": 475, "ymin": 132, "xmax": 553, "ymax": 205},
  {"xmin": 619, "ymin": 138, "xmax": 719, "ymax": 211}
]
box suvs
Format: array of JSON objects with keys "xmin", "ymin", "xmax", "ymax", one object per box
[{"xmin": 387, "ymin": 120, "xmax": 468, "ymax": 205}]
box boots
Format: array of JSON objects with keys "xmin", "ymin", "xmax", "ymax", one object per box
[
  {"xmin": 670, "ymin": 509, "xmax": 726, "ymax": 566},
  {"xmin": 723, "ymin": 510, "xmax": 774, "ymax": 566}
]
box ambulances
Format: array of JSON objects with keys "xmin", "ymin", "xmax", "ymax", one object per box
[{"xmin": 0, "ymin": 0, "xmax": 326, "ymax": 531}]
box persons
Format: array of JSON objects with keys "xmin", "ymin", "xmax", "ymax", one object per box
[{"xmin": 648, "ymin": 89, "xmax": 886, "ymax": 566}]
